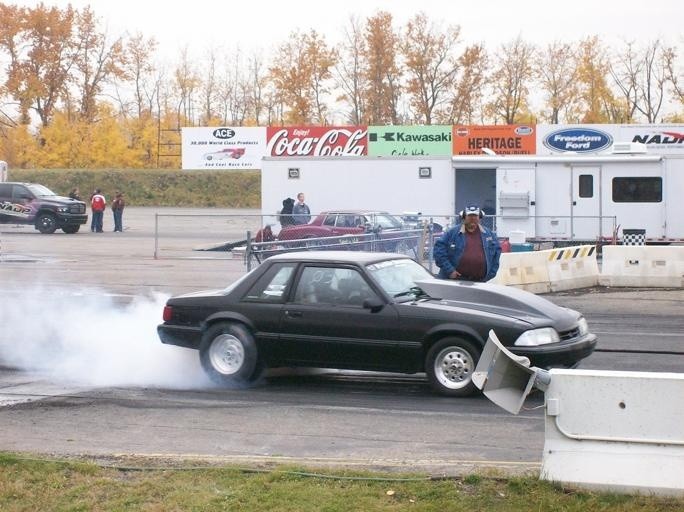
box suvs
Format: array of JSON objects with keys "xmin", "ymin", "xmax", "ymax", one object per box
[{"xmin": 0, "ymin": 181, "xmax": 89, "ymax": 234}]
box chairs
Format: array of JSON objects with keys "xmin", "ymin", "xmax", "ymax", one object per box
[{"xmin": 308, "ymin": 276, "xmax": 356, "ymax": 307}]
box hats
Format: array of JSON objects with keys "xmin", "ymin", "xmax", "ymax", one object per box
[{"xmin": 464, "ymin": 202, "xmax": 481, "ymax": 215}]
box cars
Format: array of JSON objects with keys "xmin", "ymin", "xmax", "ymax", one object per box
[
  {"xmin": 277, "ymin": 208, "xmax": 443, "ymax": 258},
  {"xmin": 156, "ymin": 249, "xmax": 598, "ymax": 399}
]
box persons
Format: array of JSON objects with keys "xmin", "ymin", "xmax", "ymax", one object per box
[
  {"xmin": 70, "ymin": 188, "xmax": 79, "ymax": 200},
  {"xmin": 92, "ymin": 189, "xmax": 105, "ymax": 234},
  {"xmin": 292, "ymin": 192, "xmax": 312, "ymax": 224},
  {"xmin": 90, "ymin": 191, "xmax": 97, "ymax": 231},
  {"xmin": 111, "ymin": 189, "xmax": 124, "ymax": 232},
  {"xmin": 433, "ymin": 202, "xmax": 505, "ymax": 285},
  {"xmin": 480, "ymin": 200, "xmax": 496, "ymax": 231},
  {"xmin": 281, "ymin": 198, "xmax": 294, "ymax": 227}
]
